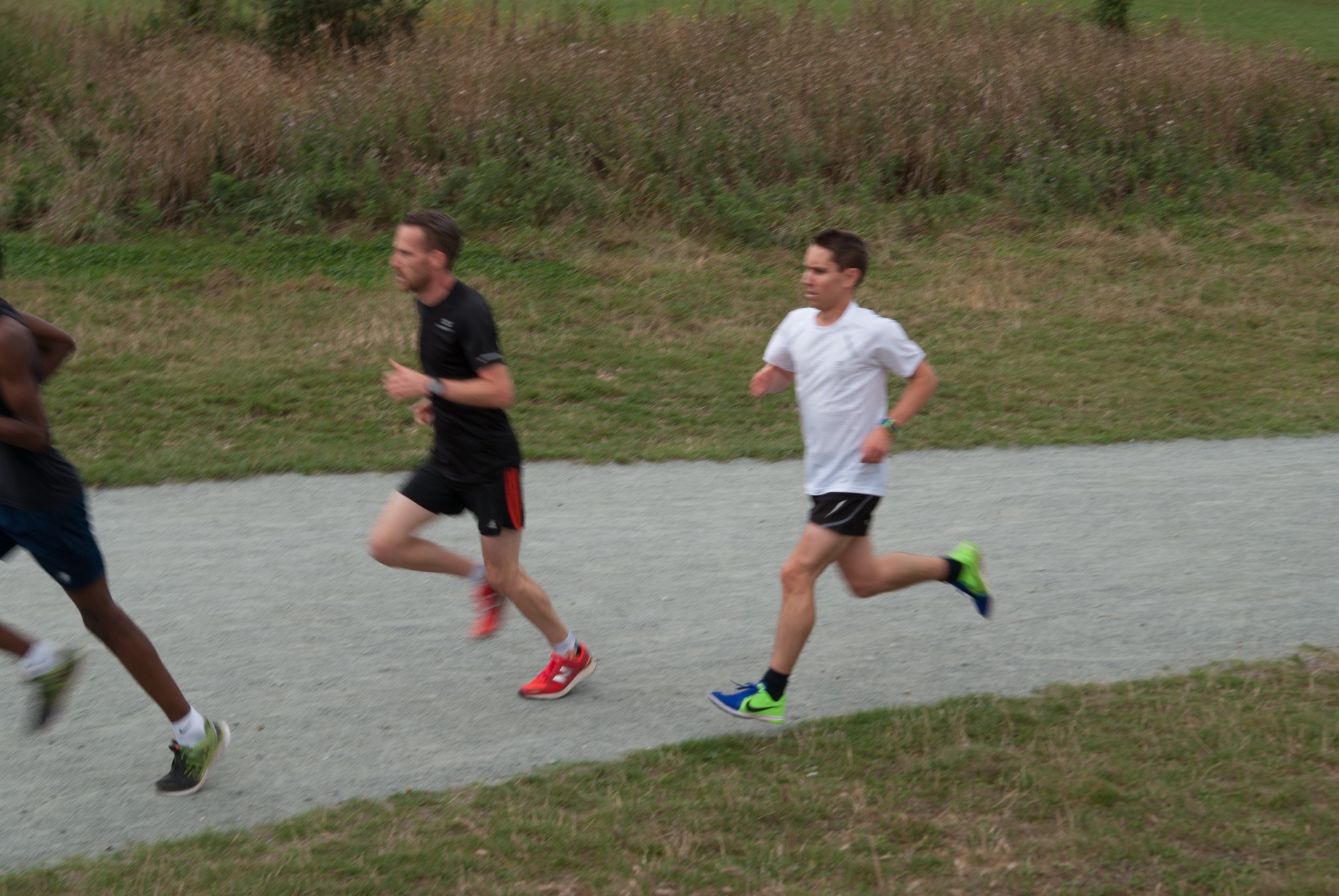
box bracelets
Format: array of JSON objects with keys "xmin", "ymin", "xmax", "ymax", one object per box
[
  {"xmin": 429, "ymin": 377, "xmax": 442, "ymax": 401},
  {"xmin": 880, "ymin": 417, "xmax": 900, "ymax": 436}
]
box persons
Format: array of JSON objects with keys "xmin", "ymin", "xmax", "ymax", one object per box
[
  {"xmin": 707, "ymin": 229, "xmax": 994, "ymax": 723},
  {"xmin": 365, "ymin": 209, "xmax": 598, "ymax": 699},
  {"xmin": 0, "ymin": 295, "xmax": 230, "ymax": 793}
]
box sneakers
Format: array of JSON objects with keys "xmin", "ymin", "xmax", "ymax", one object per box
[
  {"xmin": 470, "ymin": 579, "xmax": 504, "ymax": 634},
  {"xmin": 943, "ymin": 541, "xmax": 991, "ymax": 619},
  {"xmin": 155, "ymin": 718, "xmax": 231, "ymax": 794},
  {"xmin": 27, "ymin": 650, "xmax": 76, "ymax": 730},
  {"xmin": 708, "ymin": 679, "xmax": 786, "ymax": 726},
  {"xmin": 517, "ymin": 641, "xmax": 596, "ymax": 701}
]
